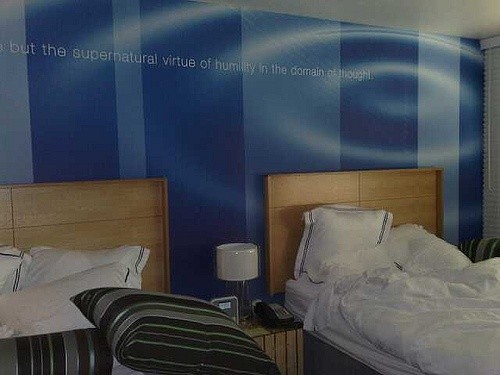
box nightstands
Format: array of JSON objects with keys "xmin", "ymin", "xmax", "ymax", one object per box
[{"xmin": 239, "ymin": 313, "xmax": 306, "ymax": 375}]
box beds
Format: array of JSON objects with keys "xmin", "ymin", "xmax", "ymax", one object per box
[
  {"xmin": 262, "ymin": 166, "xmax": 500, "ymax": 375},
  {"xmin": 0, "ymin": 177, "xmax": 173, "ymax": 375}
]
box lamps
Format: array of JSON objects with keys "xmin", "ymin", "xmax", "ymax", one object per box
[{"xmin": 213, "ymin": 241, "xmax": 262, "ymax": 329}]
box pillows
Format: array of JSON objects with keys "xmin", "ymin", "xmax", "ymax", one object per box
[
  {"xmin": 387, "ymin": 223, "xmax": 439, "ymax": 271},
  {"xmin": 22, "ymin": 245, "xmax": 150, "ymax": 311},
  {"xmin": 2, "ymin": 262, "xmax": 142, "ymax": 336},
  {"xmin": 293, "ymin": 203, "xmax": 393, "ymax": 286},
  {"xmin": 1, "ymin": 245, "xmax": 26, "ymax": 295},
  {"xmin": 1, "ymin": 327, "xmax": 113, "ymax": 375},
  {"xmin": 69, "ymin": 287, "xmax": 282, "ymax": 375},
  {"xmin": 458, "ymin": 238, "xmax": 499, "ymax": 263}
]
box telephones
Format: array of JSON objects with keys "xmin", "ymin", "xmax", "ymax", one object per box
[{"xmin": 254, "ymin": 302, "xmax": 295, "ymax": 326}]
